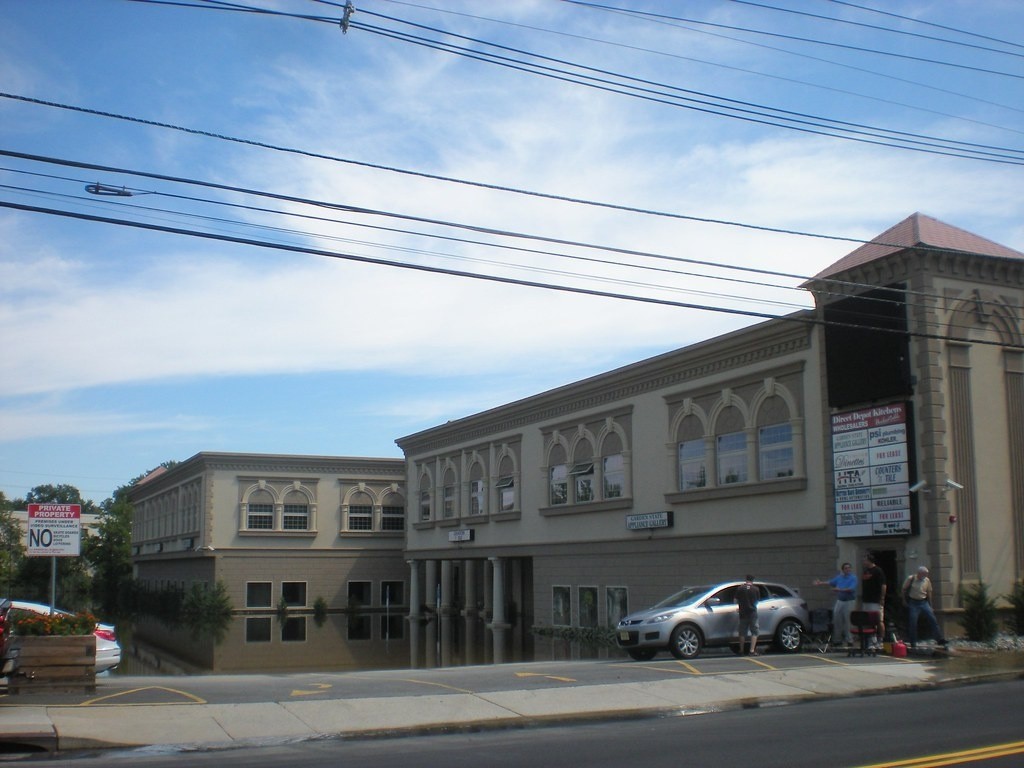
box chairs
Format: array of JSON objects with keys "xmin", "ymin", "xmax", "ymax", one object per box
[{"xmin": 846, "ymin": 611, "xmax": 879, "ymax": 658}]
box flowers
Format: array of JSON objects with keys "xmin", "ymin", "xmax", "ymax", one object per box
[{"xmin": 8, "ymin": 606, "xmax": 98, "ymax": 636}]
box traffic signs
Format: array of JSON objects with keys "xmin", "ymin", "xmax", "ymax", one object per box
[{"xmin": 26, "ymin": 503, "xmax": 82, "ymax": 558}]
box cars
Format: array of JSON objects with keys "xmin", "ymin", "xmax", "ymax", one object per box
[
  {"xmin": 1, "ymin": 599, "xmax": 122, "ymax": 677},
  {"xmin": 614, "ymin": 579, "xmax": 813, "ymax": 660}
]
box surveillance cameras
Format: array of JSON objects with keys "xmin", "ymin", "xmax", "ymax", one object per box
[
  {"xmin": 195, "ymin": 546, "xmax": 215, "ymax": 552},
  {"xmin": 909, "ymin": 479, "xmax": 932, "ymax": 494},
  {"xmin": 941, "ymin": 478, "xmax": 964, "ymax": 494}
]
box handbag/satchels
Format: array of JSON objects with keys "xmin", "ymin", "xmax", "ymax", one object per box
[{"xmin": 904, "ymin": 591, "xmax": 910, "ymax": 604}]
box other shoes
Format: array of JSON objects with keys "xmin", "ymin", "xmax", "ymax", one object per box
[
  {"xmin": 848, "ymin": 642, "xmax": 852, "ymax": 646},
  {"xmin": 739, "ymin": 651, "xmax": 749, "ymax": 657},
  {"xmin": 749, "ymin": 650, "xmax": 761, "ymax": 656},
  {"xmin": 938, "ymin": 640, "xmax": 949, "ymax": 646},
  {"xmin": 832, "ymin": 641, "xmax": 841, "ymax": 646}
]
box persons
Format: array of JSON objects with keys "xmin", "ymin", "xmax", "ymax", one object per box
[
  {"xmin": 900, "ymin": 566, "xmax": 950, "ymax": 651},
  {"xmin": 861, "ymin": 554, "xmax": 887, "ymax": 653},
  {"xmin": 811, "ymin": 562, "xmax": 857, "ymax": 646},
  {"xmin": 734, "ymin": 574, "xmax": 761, "ymax": 657}
]
